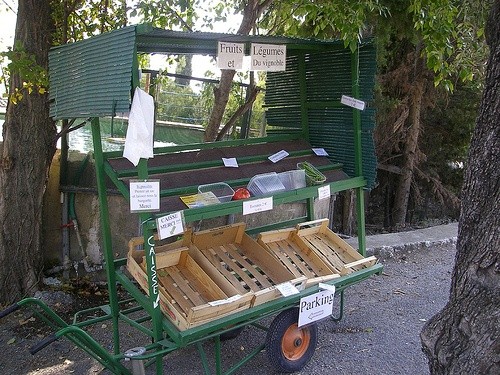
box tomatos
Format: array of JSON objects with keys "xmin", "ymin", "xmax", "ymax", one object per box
[{"xmin": 232, "ymin": 188, "xmax": 249, "ymax": 200}]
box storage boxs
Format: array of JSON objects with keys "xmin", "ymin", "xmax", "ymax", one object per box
[
  {"xmin": 256, "ymin": 227, "xmax": 341, "ymax": 288},
  {"xmin": 195, "ymin": 182, "xmax": 235, "ymax": 207},
  {"xmin": 297, "ymin": 218, "xmax": 378, "ymax": 278},
  {"xmin": 246, "ymin": 161, "xmax": 326, "ymax": 197},
  {"xmin": 192, "ymin": 222, "xmax": 308, "ymax": 306},
  {"xmin": 127, "ymin": 232, "xmax": 255, "ymax": 331}
]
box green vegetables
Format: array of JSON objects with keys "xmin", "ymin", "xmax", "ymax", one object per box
[{"xmin": 301, "ymin": 161, "xmax": 321, "ymax": 187}]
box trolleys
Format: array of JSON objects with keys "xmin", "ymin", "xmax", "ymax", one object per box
[{"xmin": 1, "ymin": 17, "xmax": 384, "ymax": 375}]
